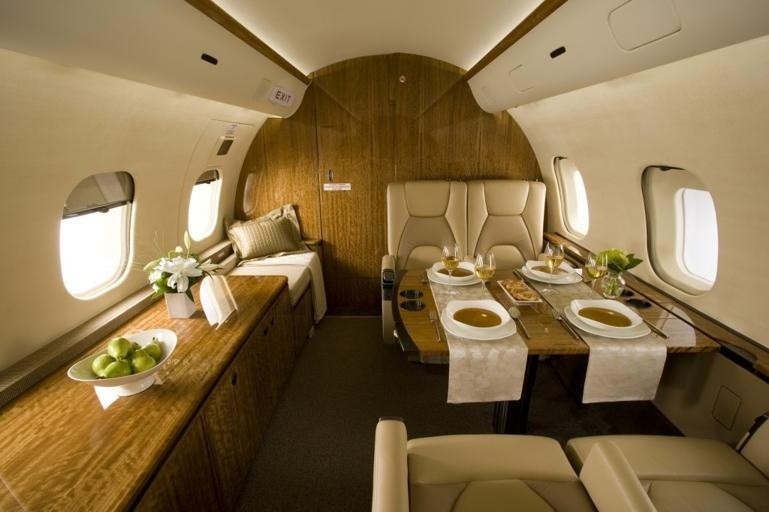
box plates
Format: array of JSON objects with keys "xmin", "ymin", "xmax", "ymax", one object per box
[
  {"xmin": 440, "ymin": 308, "xmax": 517, "ymax": 341},
  {"xmin": 497, "ymin": 280, "xmax": 544, "ymax": 305},
  {"xmin": 563, "ymin": 305, "xmax": 651, "ymax": 339},
  {"xmin": 426, "ymin": 268, "xmax": 482, "ymax": 286},
  {"xmin": 521, "ymin": 266, "xmax": 583, "ymax": 284}
]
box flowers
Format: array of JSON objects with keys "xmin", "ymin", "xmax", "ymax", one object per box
[
  {"xmin": 595, "ymin": 248, "xmax": 643, "ymax": 272},
  {"xmin": 142, "ymin": 230, "xmax": 224, "ymax": 293}
]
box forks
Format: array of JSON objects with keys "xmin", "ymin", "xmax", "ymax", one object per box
[
  {"xmin": 551, "ymin": 309, "xmax": 579, "ymax": 340},
  {"xmin": 429, "ymin": 311, "xmax": 441, "ymax": 343}
]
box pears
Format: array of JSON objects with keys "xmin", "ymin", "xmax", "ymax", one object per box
[{"xmin": 91, "ymin": 337, "xmax": 161, "ymax": 378}]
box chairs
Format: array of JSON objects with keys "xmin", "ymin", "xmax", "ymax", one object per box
[
  {"xmin": 370, "ymin": 415, "xmax": 661, "ymax": 511},
  {"xmin": 566, "ymin": 410, "xmax": 769, "ymax": 512},
  {"xmin": 468, "ymin": 180, "xmax": 547, "ymax": 270},
  {"xmin": 382, "ymin": 180, "xmax": 468, "ymax": 346}
]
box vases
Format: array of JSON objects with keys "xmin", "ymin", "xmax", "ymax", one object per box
[
  {"xmin": 164, "ymin": 291, "xmax": 197, "ymax": 318},
  {"xmin": 601, "ymin": 270, "xmax": 626, "ymax": 299}
]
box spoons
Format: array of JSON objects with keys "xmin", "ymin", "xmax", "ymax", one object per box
[{"xmin": 509, "ymin": 307, "xmax": 532, "ymax": 338}]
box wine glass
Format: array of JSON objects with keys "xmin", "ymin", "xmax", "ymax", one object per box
[
  {"xmin": 586, "ymin": 251, "xmax": 607, "ymax": 298},
  {"xmin": 441, "ymin": 245, "xmax": 462, "ymax": 295},
  {"xmin": 65, "ymin": 327, "xmax": 183, "ymax": 396},
  {"xmin": 474, "ymin": 253, "xmax": 497, "ymax": 299},
  {"xmin": 542, "ymin": 242, "xmax": 564, "ymax": 295}
]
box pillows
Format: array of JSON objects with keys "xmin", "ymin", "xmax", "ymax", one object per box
[{"xmin": 223, "ymin": 204, "xmax": 312, "ymax": 267}]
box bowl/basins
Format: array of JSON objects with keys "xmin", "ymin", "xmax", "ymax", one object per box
[
  {"xmin": 446, "ymin": 300, "xmax": 511, "ymax": 330},
  {"xmin": 526, "ymin": 260, "xmax": 576, "ymax": 279},
  {"xmin": 432, "ymin": 261, "xmax": 475, "ymax": 280},
  {"xmin": 570, "ymin": 298, "xmax": 643, "ymax": 329}
]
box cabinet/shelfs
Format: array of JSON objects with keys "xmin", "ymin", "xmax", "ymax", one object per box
[{"xmin": 0, "ymin": 275, "xmax": 313, "ymax": 511}]
